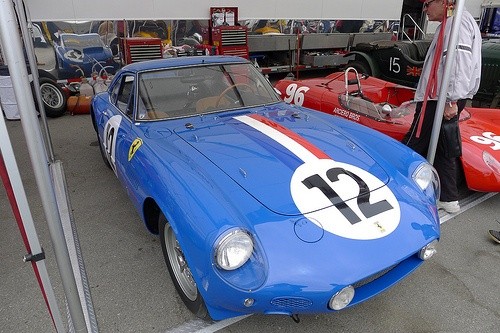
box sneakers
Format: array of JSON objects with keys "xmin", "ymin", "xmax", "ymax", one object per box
[
  {"xmin": 438, "ymin": 201, "xmax": 461, "ymax": 213},
  {"xmin": 488, "ymin": 229, "xmax": 500, "ymax": 244}
]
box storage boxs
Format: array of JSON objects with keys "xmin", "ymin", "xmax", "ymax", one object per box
[{"xmin": 302, "ymin": 54, "xmax": 355, "ymax": 66}]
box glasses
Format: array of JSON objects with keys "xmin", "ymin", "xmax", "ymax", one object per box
[{"xmin": 423, "ymin": 0, "xmax": 436, "ymax": 8}]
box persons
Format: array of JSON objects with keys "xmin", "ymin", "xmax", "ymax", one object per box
[{"xmin": 401, "ymin": 0, "xmax": 482, "ymax": 213}]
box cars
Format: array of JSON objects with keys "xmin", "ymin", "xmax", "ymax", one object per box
[{"xmin": 19, "ymin": 23, "xmax": 57, "ymax": 82}]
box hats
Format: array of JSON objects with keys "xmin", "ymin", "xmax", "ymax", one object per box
[{"xmin": 268, "ymin": 19, "xmax": 279, "ymax": 24}]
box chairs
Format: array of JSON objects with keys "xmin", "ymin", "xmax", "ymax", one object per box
[
  {"xmin": 395, "ymin": 41, "xmax": 433, "ymax": 65},
  {"xmin": 62, "ymin": 39, "xmax": 101, "ymax": 48},
  {"xmin": 345, "ymin": 67, "xmax": 399, "ymax": 118},
  {"xmin": 196, "ymin": 96, "xmax": 231, "ymax": 113},
  {"xmin": 141, "ymin": 111, "xmax": 169, "ymax": 119}
]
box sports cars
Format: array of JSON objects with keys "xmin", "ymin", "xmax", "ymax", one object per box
[
  {"xmin": 54, "ymin": 33, "xmax": 114, "ymax": 80},
  {"xmin": 91, "ymin": 55, "xmax": 441, "ymax": 324},
  {"xmin": 343, "ymin": 13, "xmax": 500, "ymax": 108},
  {"xmin": 272, "ymin": 66, "xmax": 500, "ymax": 193}
]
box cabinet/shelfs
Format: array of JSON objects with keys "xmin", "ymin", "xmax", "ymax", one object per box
[{"xmin": 247, "ymin": 32, "xmax": 392, "ymax": 75}]
